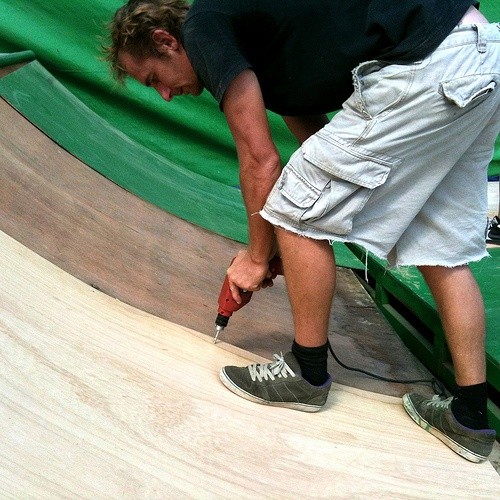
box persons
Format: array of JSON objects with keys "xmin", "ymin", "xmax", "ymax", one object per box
[{"xmin": 102, "ymin": 0, "xmax": 500, "ymax": 465}]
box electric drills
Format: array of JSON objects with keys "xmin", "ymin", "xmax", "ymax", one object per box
[{"xmin": 212, "ymin": 257, "xmax": 288, "ymax": 345}]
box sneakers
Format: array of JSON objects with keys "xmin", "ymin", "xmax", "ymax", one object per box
[
  {"xmin": 219, "ymin": 352, "xmax": 333, "ymax": 412},
  {"xmin": 402, "ymin": 393, "xmax": 496, "ymax": 463}
]
